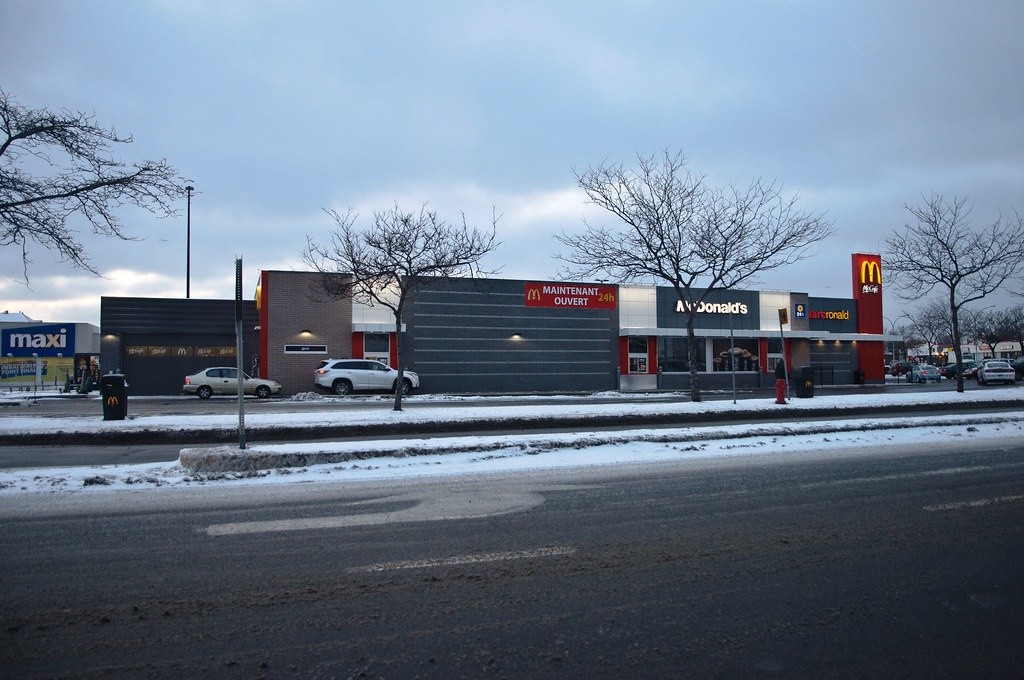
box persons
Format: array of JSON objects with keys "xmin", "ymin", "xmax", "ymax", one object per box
[
  {"xmin": 251, "ymin": 352, "xmax": 259, "ymax": 376},
  {"xmin": 774, "ymin": 359, "xmax": 790, "ymax": 383}
]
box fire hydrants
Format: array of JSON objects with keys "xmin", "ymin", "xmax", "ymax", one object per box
[{"xmin": 775, "ymin": 378, "xmax": 786, "ymax": 404}]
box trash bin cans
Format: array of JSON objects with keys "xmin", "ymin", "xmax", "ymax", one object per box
[
  {"xmin": 855, "ymin": 370, "xmax": 865, "ymax": 384},
  {"xmin": 794, "ymin": 367, "xmax": 813, "ymax": 398},
  {"xmin": 102, "ymin": 374, "xmax": 128, "ymax": 420}
]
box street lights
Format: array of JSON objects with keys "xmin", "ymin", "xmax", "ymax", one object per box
[
  {"xmin": 185, "ymin": 185, "xmax": 195, "ymax": 299},
  {"xmin": 958, "ymin": 304, "xmax": 996, "ymax": 362},
  {"xmin": 883, "ymin": 313, "xmax": 910, "ymax": 363}
]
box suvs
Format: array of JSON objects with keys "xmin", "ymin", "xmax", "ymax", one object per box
[{"xmin": 314, "ymin": 358, "xmax": 420, "ymax": 395}]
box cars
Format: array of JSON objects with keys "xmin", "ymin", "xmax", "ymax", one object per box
[
  {"xmin": 890, "ymin": 361, "xmax": 916, "ymax": 376},
  {"xmin": 940, "ymin": 362, "xmax": 978, "ymax": 379},
  {"xmin": 906, "ymin": 365, "xmax": 941, "ymax": 384},
  {"xmin": 182, "ymin": 366, "xmax": 282, "ymax": 399},
  {"xmin": 962, "ymin": 356, "xmax": 1024, "ymax": 381},
  {"xmin": 977, "ymin": 362, "xmax": 1016, "ymax": 386}
]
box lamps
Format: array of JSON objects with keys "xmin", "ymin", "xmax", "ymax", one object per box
[
  {"xmin": 301, "ymin": 330, "xmax": 311, "ymax": 333},
  {"xmin": 512, "ymin": 332, "xmax": 522, "ymax": 336}
]
